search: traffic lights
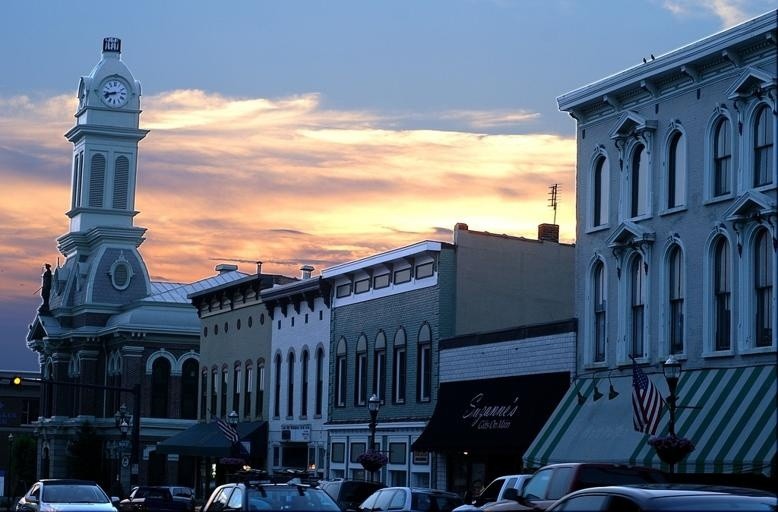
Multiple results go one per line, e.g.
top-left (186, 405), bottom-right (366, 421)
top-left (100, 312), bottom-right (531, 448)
top-left (11, 376), bottom-right (22, 387)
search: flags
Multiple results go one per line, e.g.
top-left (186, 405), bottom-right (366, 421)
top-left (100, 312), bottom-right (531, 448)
top-left (632, 358), bottom-right (662, 436)
top-left (208, 408), bottom-right (239, 445)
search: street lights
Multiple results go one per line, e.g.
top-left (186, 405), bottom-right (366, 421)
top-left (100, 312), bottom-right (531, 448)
top-left (368, 394), bottom-right (381, 480)
top-left (662, 354), bottom-right (681, 467)
top-left (228, 409), bottom-right (239, 480)
top-left (120, 420), bottom-right (129, 489)
